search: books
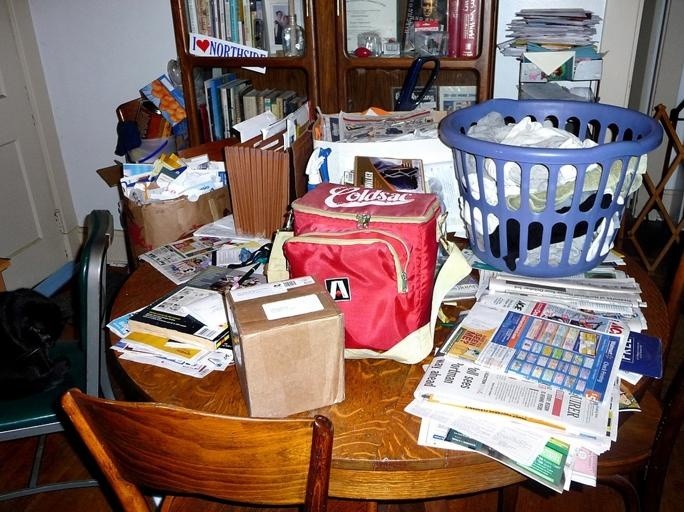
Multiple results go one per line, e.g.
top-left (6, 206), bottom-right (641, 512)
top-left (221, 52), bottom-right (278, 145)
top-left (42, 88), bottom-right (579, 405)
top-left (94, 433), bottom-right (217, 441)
top-left (194, 71), bottom-right (308, 141)
top-left (182, 0), bottom-right (266, 58)
top-left (399, 0), bottom-right (483, 60)
top-left (118, 264), bottom-right (268, 366)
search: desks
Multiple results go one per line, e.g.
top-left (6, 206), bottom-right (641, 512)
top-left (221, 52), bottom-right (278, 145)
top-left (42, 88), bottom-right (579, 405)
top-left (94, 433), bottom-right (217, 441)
top-left (108, 227), bottom-right (670, 501)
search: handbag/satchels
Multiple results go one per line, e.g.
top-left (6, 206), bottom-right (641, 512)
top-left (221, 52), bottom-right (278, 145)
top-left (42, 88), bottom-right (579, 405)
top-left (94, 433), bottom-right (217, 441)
top-left (265, 182), bottom-right (473, 367)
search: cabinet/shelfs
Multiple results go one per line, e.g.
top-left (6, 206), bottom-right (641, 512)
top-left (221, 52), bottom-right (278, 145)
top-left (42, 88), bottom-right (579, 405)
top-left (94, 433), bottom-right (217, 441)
top-left (169, 0), bottom-right (500, 145)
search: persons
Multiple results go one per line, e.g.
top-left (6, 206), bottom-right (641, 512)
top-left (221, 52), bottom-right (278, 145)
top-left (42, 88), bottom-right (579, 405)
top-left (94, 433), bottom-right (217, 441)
top-left (273, 10), bottom-right (283, 44)
top-left (283, 16), bottom-right (289, 25)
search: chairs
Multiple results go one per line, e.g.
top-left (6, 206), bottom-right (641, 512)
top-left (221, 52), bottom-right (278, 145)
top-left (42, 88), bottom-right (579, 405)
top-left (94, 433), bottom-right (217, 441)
top-left (61, 388), bottom-right (334, 512)
top-left (0, 208), bottom-right (115, 502)
